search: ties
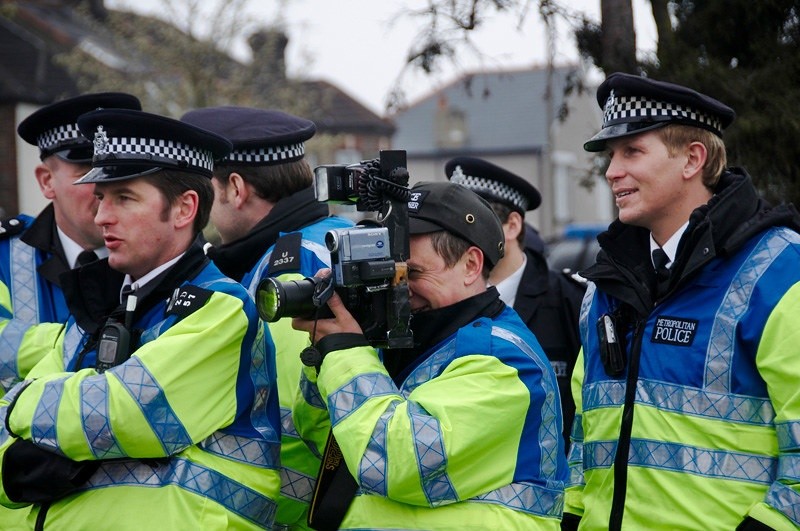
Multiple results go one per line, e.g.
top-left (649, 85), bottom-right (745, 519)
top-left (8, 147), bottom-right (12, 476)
top-left (652, 249), bottom-right (672, 295)
top-left (78, 250), bottom-right (98, 266)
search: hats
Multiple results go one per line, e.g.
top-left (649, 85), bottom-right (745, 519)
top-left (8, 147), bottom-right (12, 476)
top-left (17, 92), bottom-right (143, 164)
top-left (582, 71), bottom-right (736, 152)
top-left (409, 181), bottom-right (506, 267)
top-left (445, 156), bottom-right (542, 218)
top-left (180, 106), bottom-right (317, 165)
top-left (72, 109), bottom-right (234, 188)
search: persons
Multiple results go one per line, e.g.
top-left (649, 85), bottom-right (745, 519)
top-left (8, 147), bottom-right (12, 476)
top-left (0, 109), bottom-right (282, 531)
top-left (560, 71), bottom-right (800, 531)
top-left (0, 90), bottom-right (143, 445)
top-left (444, 157), bottom-right (584, 458)
top-left (178, 107), bottom-right (332, 531)
top-left (292, 182), bottom-right (571, 531)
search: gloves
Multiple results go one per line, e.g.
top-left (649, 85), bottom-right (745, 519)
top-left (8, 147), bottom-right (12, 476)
top-left (8, 437), bottom-right (95, 506)
